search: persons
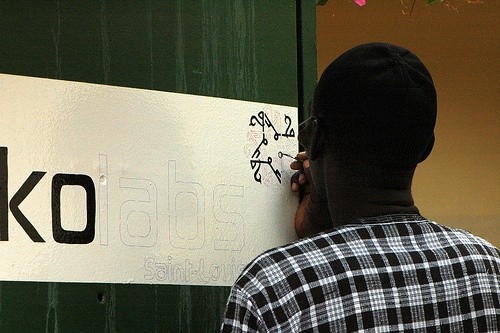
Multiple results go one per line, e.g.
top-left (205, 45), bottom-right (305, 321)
top-left (219, 42), bottom-right (500, 333)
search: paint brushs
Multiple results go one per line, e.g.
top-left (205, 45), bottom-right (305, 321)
top-left (283, 154), bottom-right (304, 163)
